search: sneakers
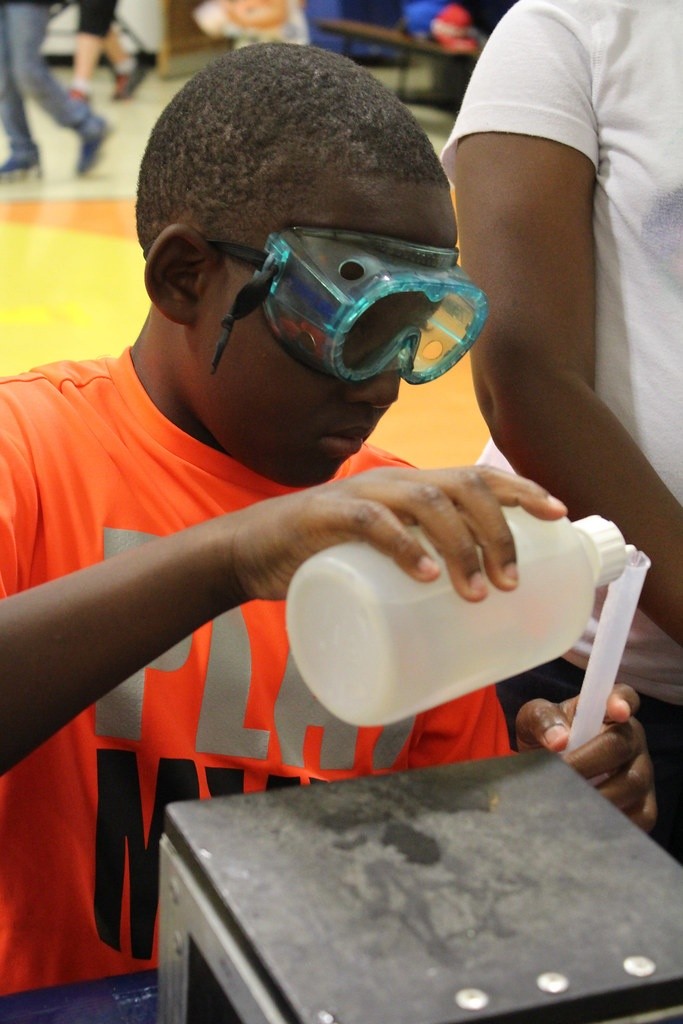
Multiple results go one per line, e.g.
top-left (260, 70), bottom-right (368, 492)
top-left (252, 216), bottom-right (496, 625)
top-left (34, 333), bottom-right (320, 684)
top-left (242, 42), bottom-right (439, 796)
top-left (76, 119), bottom-right (114, 174)
top-left (0, 147), bottom-right (40, 184)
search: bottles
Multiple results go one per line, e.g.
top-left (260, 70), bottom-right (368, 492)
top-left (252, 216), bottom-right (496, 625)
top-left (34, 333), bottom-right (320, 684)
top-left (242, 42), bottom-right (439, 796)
top-left (284, 504), bottom-right (637, 729)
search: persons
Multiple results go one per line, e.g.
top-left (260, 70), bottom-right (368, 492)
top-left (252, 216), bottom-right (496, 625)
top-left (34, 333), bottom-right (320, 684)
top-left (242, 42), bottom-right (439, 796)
top-left (0, 45), bottom-right (661, 1024)
top-left (442, 1), bottom-right (683, 861)
top-left (0, 1), bottom-right (113, 180)
top-left (66, 2), bottom-right (150, 114)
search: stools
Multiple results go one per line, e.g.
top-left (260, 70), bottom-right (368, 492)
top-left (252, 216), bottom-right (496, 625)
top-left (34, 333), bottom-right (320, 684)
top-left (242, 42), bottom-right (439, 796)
top-left (156, 749), bottom-right (683, 1024)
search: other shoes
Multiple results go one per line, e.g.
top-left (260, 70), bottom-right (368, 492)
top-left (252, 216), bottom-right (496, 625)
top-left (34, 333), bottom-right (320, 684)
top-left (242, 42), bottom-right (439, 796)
top-left (112, 53), bottom-right (156, 101)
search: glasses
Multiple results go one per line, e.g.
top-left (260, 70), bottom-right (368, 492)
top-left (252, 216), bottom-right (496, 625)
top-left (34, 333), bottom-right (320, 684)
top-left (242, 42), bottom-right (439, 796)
top-left (202, 221), bottom-right (491, 386)
